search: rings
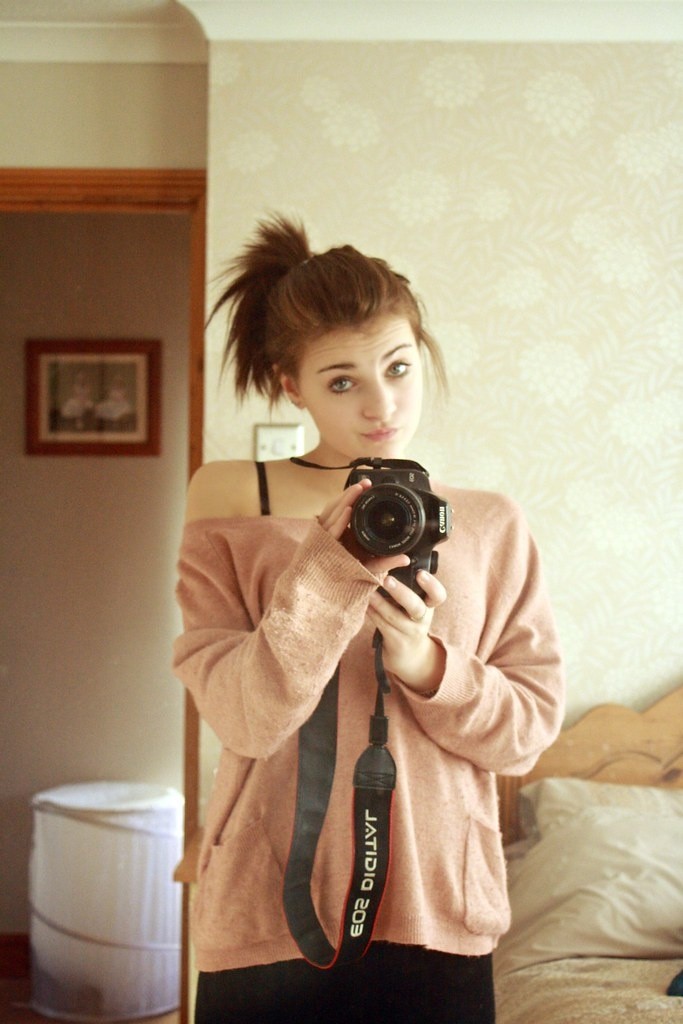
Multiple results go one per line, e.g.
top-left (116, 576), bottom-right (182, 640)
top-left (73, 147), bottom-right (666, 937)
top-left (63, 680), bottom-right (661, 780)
top-left (411, 610), bottom-right (427, 622)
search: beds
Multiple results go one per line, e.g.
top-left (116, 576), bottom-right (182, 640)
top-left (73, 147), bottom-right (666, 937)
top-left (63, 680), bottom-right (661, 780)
top-left (491, 683), bottom-right (682, 1023)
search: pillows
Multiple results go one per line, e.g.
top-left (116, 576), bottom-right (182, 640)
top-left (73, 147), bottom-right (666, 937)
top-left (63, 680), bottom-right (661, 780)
top-left (515, 777), bottom-right (682, 849)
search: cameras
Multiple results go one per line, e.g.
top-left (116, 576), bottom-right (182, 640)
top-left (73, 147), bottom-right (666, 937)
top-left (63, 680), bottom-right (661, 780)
top-left (344, 458), bottom-right (450, 604)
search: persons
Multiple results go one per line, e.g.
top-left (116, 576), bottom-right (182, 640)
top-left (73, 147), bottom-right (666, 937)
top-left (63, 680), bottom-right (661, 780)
top-left (173, 217), bottom-right (566, 1024)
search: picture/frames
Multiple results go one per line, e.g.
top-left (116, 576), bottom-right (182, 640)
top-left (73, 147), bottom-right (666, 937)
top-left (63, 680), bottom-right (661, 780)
top-left (25, 339), bottom-right (164, 456)
